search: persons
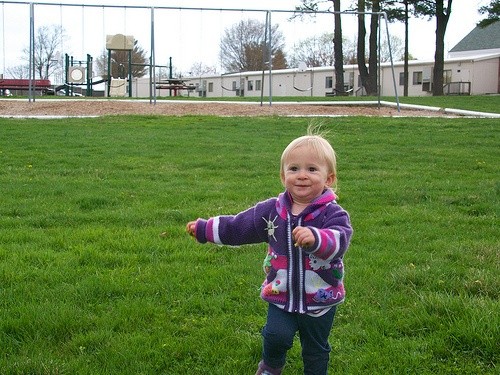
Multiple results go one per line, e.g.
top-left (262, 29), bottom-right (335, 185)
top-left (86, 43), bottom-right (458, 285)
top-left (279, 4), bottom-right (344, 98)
top-left (187, 119), bottom-right (353, 375)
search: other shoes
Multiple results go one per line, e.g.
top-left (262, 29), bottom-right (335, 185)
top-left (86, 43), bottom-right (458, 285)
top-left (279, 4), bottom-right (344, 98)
top-left (256, 360), bottom-right (283, 375)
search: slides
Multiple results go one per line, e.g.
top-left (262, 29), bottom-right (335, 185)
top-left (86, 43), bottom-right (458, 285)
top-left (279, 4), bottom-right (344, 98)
top-left (55, 83), bottom-right (66, 92)
